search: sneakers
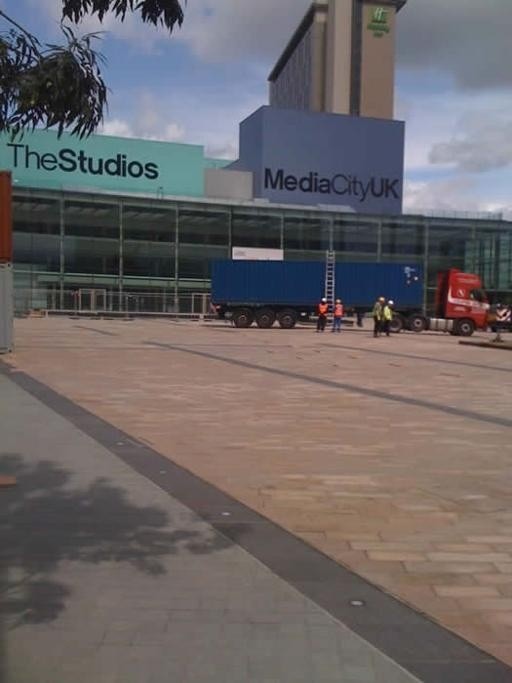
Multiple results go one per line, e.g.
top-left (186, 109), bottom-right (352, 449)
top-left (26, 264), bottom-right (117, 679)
top-left (315, 328), bottom-right (325, 333)
top-left (331, 328), bottom-right (342, 333)
top-left (373, 330), bottom-right (390, 337)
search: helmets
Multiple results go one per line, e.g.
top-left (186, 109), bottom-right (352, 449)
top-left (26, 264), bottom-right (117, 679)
top-left (387, 299), bottom-right (394, 305)
top-left (321, 297), bottom-right (328, 303)
top-left (379, 296), bottom-right (385, 301)
top-left (336, 299), bottom-right (342, 304)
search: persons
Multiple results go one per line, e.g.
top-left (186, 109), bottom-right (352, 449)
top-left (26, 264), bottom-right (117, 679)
top-left (316, 298), bottom-right (329, 330)
top-left (332, 299), bottom-right (343, 332)
top-left (380, 300), bottom-right (394, 336)
top-left (372, 297), bottom-right (385, 336)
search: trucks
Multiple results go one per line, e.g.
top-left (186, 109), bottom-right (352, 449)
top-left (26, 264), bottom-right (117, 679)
top-left (210, 259), bottom-right (490, 336)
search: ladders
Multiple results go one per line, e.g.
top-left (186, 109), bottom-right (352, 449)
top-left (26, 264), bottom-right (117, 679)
top-left (324, 249), bottom-right (335, 326)
